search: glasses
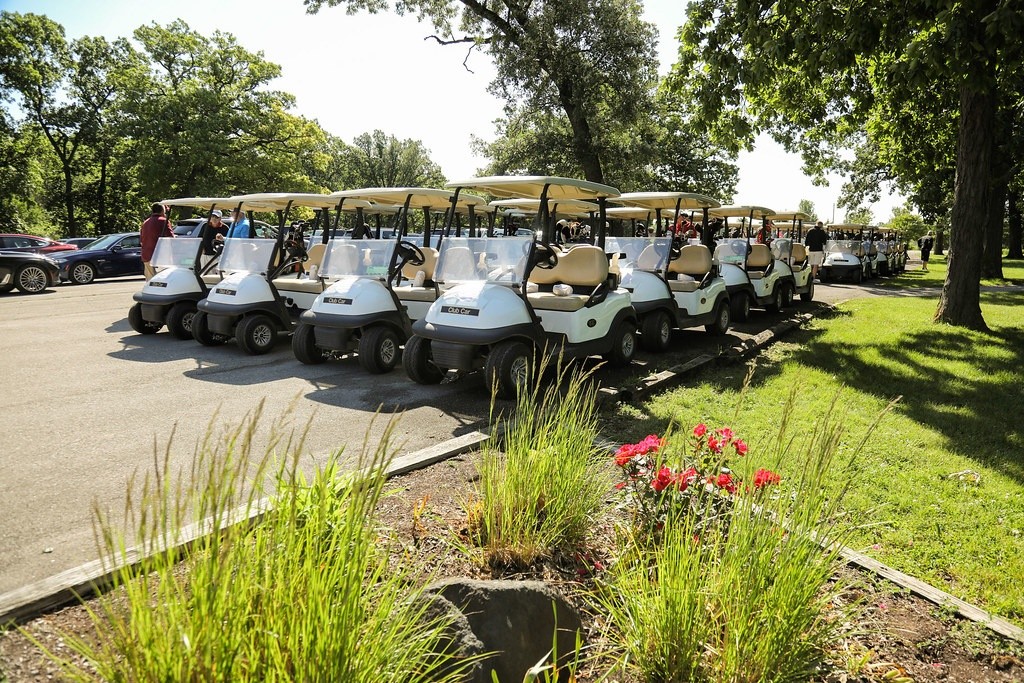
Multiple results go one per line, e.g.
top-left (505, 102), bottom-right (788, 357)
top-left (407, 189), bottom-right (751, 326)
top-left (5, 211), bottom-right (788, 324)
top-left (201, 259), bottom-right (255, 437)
top-left (211, 214), bottom-right (219, 218)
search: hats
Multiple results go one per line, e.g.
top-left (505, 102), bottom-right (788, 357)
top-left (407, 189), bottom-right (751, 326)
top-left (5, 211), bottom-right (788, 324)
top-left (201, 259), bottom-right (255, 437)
top-left (228, 208), bottom-right (247, 213)
top-left (212, 209), bottom-right (223, 217)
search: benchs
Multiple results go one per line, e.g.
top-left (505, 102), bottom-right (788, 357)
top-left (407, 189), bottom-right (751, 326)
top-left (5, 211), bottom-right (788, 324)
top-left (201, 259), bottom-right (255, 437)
top-left (197, 242), bottom-right (904, 311)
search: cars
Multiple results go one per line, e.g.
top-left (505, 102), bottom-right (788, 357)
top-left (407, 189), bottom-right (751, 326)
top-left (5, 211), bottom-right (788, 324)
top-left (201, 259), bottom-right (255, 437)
top-left (0, 249), bottom-right (62, 295)
top-left (0, 234), bottom-right (78, 254)
top-left (45, 232), bottom-right (145, 285)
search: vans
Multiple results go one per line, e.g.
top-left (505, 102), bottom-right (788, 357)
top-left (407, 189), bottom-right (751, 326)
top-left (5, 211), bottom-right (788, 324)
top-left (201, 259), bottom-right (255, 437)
top-left (172, 218), bottom-right (287, 239)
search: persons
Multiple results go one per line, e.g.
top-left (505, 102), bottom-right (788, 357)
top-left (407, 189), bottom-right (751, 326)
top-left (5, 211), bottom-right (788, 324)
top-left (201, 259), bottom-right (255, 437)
top-left (918, 230), bottom-right (934, 271)
top-left (140, 202), bottom-right (176, 284)
top-left (668, 214), bottom-right (831, 284)
top-left (196, 208), bottom-right (251, 276)
